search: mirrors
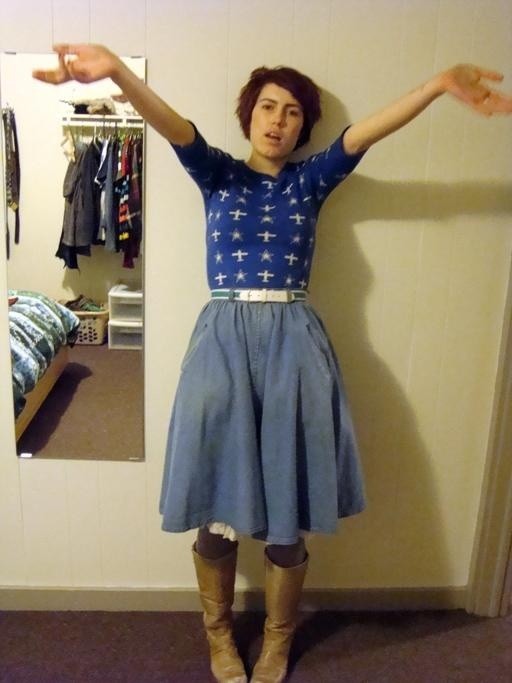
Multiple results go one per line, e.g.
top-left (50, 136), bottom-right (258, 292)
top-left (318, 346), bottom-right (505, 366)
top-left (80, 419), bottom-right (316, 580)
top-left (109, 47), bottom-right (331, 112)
top-left (0, 50), bottom-right (146, 463)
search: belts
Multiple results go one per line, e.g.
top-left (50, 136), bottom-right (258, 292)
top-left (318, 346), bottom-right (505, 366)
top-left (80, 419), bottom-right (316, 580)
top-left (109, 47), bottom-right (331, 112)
top-left (211, 289), bottom-right (308, 303)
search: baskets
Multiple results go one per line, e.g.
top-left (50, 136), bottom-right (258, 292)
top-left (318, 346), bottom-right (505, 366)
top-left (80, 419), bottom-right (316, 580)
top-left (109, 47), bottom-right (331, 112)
top-left (68, 307), bottom-right (109, 345)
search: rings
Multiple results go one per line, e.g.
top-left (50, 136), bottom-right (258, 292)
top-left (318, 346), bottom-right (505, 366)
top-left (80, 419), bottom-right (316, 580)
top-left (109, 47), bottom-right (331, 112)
top-left (482, 95), bottom-right (490, 103)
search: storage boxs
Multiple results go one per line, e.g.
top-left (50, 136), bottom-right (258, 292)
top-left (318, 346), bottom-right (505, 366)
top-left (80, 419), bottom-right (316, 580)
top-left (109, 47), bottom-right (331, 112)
top-left (108, 279), bottom-right (143, 350)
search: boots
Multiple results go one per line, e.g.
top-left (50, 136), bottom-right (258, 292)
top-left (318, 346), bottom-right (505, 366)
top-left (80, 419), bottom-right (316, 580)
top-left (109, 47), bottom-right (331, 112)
top-left (192, 541), bottom-right (247, 682)
top-left (250, 546), bottom-right (309, 683)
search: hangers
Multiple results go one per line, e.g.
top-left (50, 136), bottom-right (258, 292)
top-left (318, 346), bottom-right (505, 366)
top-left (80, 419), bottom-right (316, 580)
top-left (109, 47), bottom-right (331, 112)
top-left (67, 122), bottom-right (144, 142)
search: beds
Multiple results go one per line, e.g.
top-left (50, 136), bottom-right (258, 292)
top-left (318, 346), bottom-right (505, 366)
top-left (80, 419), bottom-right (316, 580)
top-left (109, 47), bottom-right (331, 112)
top-left (8, 288), bottom-right (81, 443)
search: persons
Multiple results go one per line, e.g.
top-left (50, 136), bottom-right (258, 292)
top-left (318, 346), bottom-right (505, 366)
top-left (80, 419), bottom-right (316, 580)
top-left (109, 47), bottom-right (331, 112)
top-left (30, 42), bottom-right (512, 683)
